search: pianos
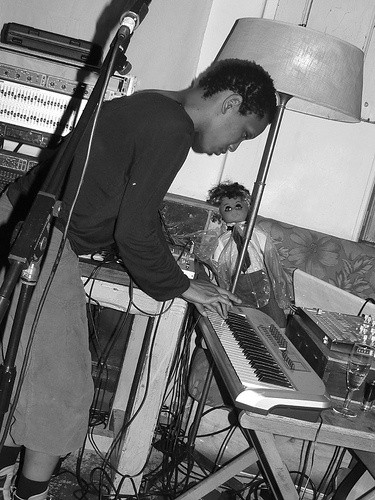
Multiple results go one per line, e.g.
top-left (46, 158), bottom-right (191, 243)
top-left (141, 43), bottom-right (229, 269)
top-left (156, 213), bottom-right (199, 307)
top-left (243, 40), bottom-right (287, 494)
top-left (191, 296), bottom-right (334, 418)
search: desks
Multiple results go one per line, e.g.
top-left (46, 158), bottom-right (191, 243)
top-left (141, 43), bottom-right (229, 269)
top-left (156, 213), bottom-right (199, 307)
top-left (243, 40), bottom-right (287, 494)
top-left (166, 321), bottom-right (375, 500)
top-left (78, 252), bottom-right (194, 500)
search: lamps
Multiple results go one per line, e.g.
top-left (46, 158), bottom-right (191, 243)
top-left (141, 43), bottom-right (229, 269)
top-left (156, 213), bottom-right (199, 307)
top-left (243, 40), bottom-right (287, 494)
top-left (138, 18), bottom-right (364, 495)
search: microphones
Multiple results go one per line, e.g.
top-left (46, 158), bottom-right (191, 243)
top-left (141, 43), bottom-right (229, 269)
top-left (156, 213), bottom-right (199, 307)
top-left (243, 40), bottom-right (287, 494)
top-left (117, 10), bottom-right (139, 39)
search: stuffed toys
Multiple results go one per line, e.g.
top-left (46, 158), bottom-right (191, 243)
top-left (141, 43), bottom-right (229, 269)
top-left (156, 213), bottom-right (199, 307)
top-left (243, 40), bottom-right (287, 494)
top-left (209, 181), bottom-right (291, 328)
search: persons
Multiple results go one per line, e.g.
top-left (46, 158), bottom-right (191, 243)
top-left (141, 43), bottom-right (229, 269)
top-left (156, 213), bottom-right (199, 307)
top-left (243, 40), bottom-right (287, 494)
top-left (0, 58), bottom-right (278, 500)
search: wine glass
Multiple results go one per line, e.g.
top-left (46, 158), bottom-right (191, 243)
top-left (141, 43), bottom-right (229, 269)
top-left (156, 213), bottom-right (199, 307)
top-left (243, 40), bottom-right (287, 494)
top-left (332, 343), bottom-right (375, 418)
